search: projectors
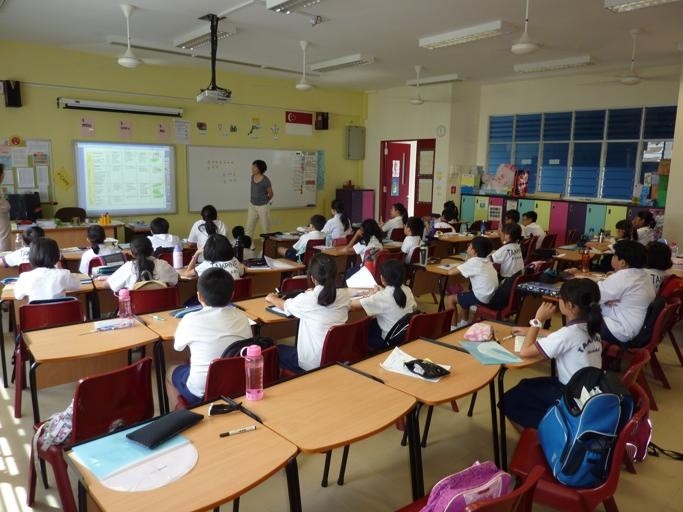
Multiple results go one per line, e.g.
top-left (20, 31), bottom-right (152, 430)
top-left (196, 89), bottom-right (232, 105)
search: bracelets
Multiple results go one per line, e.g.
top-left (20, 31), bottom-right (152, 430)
top-left (191, 255), bottom-right (198, 262)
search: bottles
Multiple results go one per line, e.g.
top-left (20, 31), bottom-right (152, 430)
top-left (588, 228), bottom-right (594, 240)
top-left (14, 232), bottom-right (23, 250)
top-left (240, 342), bottom-right (266, 401)
top-left (171, 243), bottom-right (184, 270)
top-left (111, 288), bottom-right (133, 321)
top-left (479, 217), bottom-right (486, 235)
top-left (578, 247), bottom-right (590, 273)
top-left (325, 233), bottom-right (332, 249)
top-left (105, 212), bottom-right (111, 225)
top-left (597, 231), bottom-right (604, 245)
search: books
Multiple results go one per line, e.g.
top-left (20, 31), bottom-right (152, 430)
top-left (70, 265), bottom-right (121, 284)
top-left (56, 245), bottom-right (81, 255)
top-left (246, 255), bottom-right (296, 270)
top-left (119, 240), bottom-right (135, 250)
top-left (71, 427), bottom-right (192, 482)
top-left (34, 217), bottom-right (57, 230)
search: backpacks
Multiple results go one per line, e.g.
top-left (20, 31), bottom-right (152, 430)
top-left (524, 259), bottom-right (546, 275)
top-left (625, 414), bottom-right (683, 474)
top-left (613, 295), bottom-right (666, 372)
top-left (133, 270), bottom-right (167, 291)
top-left (418, 460), bottom-right (516, 512)
top-left (385, 311), bottom-right (427, 347)
top-left (488, 263), bottom-right (536, 310)
top-left (219, 336), bottom-right (273, 358)
top-left (31, 398), bottom-right (73, 494)
top-left (537, 366), bottom-right (636, 489)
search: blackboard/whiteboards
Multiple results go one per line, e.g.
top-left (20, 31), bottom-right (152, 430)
top-left (187, 146), bottom-right (319, 214)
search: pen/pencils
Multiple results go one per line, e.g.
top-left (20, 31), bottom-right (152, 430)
top-left (503, 333), bottom-right (514, 341)
top-left (149, 315), bottom-right (165, 321)
top-left (220, 424), bottom-right (256, 437)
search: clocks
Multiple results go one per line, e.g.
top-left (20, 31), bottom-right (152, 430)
top-left (435, 125), bottom-right (446, 137)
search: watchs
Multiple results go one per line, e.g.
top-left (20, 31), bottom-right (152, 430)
top-left (529, 319), bottom-right (544, 329)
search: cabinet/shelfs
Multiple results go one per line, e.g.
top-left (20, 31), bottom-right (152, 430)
top-left (334, 188), bottom-right (374, 220)
top-left (459, 193), bottom-right (664, 251)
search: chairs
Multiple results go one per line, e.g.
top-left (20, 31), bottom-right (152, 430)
top-left (55, 207), bottom-right (85, 221)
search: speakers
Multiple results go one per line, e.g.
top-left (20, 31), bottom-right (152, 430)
top-left (3, 80), bottom-right (21, 107)
top-left (314, 111), bottom-right (329, 130)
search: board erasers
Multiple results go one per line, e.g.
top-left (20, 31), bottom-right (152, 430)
top-left (306, 203), bottom-right (316, 207)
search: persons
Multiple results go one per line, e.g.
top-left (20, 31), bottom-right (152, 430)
top-left (236, 235), bottom-right (257, 259)
top-left (166, 265), bottom-right (255, 409)
top-left (585, 212), bottom-right (674, 345)
top-left (0, 161), bottom-right (13, 253)
top-left (100, 234), bottom-right (180, 316)
top-left (264, 254), bottom-right (353, 378)
top-left (493, 276), bottom-right (606, 438)
top-left (146, 218), bottom-right (182, 252)
top-left (231, 226), bottom-right (256, 251)
top-left (275, 198), bottom-right (547, 376)
top-left (79, 223), bottom-right (128, 274)
top-left (0, 225), bottom-right (46, 266)
top-left (186, 204), bottom-right (228, 251)
top-left (184, 234), bottom-right (249, 281)
top-left (243, 158), bottom-right (275, 256)
top-left (12, 238), bottom-right (82, 303)
